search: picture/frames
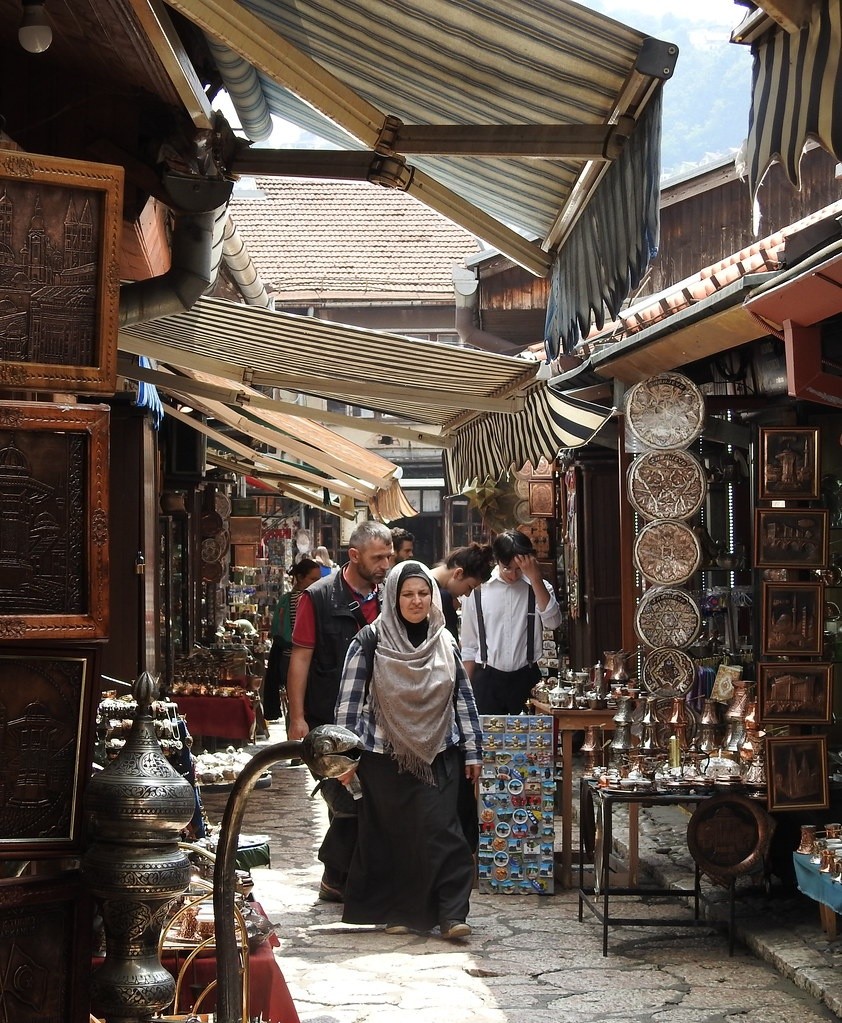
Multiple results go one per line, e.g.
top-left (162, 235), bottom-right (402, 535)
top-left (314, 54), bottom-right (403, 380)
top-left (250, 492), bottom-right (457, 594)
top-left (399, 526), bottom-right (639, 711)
top-left (0, 148), bottom-right (125, 1023)
top-left (340, 507), bottom-right (368, 546)
top-left (752, 428), bottom-right (834, 811)
top-left (528, 456), bottom-right (555, 518)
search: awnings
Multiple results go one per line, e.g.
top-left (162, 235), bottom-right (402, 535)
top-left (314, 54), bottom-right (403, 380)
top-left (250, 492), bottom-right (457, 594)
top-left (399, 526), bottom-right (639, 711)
top-left (156, 362), bottom-right (419, 523)
top-left (165, 0), bottom-right (679, 365)
top-left (730, 0), bottom-right (841, 199)
top-left (114, 297), bottom-right (615, 497)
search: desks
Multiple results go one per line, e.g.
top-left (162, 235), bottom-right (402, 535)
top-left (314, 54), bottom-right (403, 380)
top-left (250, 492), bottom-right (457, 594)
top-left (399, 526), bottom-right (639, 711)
top-left (791, 851), bottom-right (842, 937)
top-left (93, 901), bottom-right (299, 1023)
top-left (532, 698), bottom-right (750, 956)
top-left (168, 694), bottom-right (255, 753)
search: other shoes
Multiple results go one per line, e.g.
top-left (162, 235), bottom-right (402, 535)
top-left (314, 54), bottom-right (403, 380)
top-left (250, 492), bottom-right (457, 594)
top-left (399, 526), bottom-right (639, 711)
top-left (441, 919), bottom-right (473, 939)
top-left (384, 923), bottom-right (410, 935)
top-left (319, 875), bottom-right (345, 902)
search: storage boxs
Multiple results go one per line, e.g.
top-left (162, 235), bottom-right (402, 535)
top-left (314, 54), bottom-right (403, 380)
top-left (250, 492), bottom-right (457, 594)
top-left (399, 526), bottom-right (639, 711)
top-left (230, 498), bottom-right (262, 566)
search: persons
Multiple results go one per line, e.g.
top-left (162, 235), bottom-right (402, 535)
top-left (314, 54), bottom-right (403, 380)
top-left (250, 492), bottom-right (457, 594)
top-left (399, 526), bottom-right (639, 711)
top-left (314, 546), bottom-right (333, 577)
top-left (277, 558), bottom-right (321, 766)
top-left (430, 543), bottom-right (493, 641)
top-left (338, 560), bottom-right (484, 940)
top-left (461, 530), bottom-right (563, 715)
top-left (388, 528), bottom-right (415, 569)
top-left (288, 521), bottom-right (392, 903)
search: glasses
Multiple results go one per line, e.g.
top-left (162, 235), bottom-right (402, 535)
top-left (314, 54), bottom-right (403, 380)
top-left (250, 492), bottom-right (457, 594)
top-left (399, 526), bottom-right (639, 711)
top-left (499, 566), bottom-right (523, 574)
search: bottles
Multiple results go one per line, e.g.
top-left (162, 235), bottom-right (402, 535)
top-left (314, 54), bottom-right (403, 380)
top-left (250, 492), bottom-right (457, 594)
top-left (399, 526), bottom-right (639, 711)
top-left (532, 650), bottom-right (768, 787)
top-left (347, 775), bottom-right (364, 800)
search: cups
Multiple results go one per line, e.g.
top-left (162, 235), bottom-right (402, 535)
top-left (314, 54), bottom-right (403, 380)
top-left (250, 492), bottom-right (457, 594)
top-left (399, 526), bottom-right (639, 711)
top-left (796, 824), bottom-right (842, 882)
